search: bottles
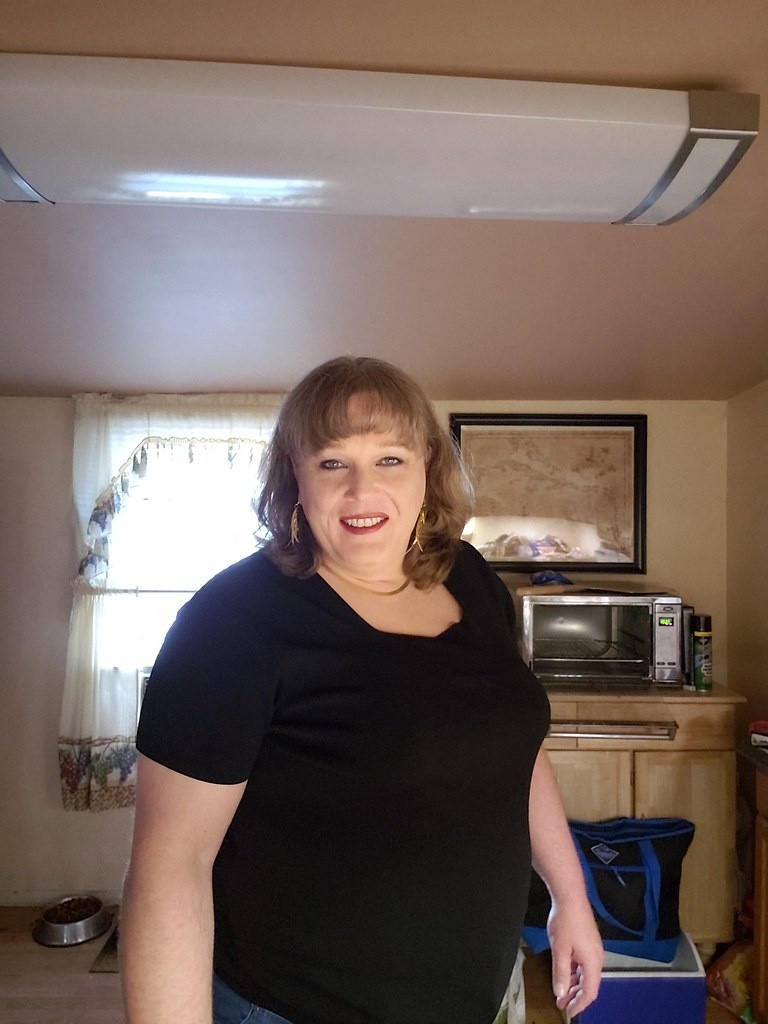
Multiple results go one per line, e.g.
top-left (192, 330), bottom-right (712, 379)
top-left (694, 616), bottom-right (714, 692)
top-left (682, 607), bottom-right (695, 685)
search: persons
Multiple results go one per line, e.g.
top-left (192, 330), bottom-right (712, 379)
top-left (118, 356), bottom-right (604, 1024)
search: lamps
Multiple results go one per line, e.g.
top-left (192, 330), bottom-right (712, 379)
top-left (0, 52), bottom-right (758, 228)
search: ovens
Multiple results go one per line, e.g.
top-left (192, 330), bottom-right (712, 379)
top-left (522, 595), bottom-right (683, 689)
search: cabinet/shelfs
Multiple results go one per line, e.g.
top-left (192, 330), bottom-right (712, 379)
top-left (529, 683), bottom-right (749, 967)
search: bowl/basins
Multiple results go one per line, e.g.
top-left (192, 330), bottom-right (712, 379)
top-left (33, 895), bottom-right (112, 947)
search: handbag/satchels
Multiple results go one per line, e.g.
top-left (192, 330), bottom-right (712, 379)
top-left (521, 816), bottom-right (696, 964)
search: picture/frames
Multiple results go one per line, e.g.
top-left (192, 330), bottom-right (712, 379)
top-left (449, 411), bottom-right (647, 575)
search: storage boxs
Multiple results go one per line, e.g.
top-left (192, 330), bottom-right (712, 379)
top-left (565, 928), bottom-right (706, 1024)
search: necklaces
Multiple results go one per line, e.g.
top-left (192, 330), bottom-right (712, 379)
top-left (320, 560), bottom-right (413, 596)
